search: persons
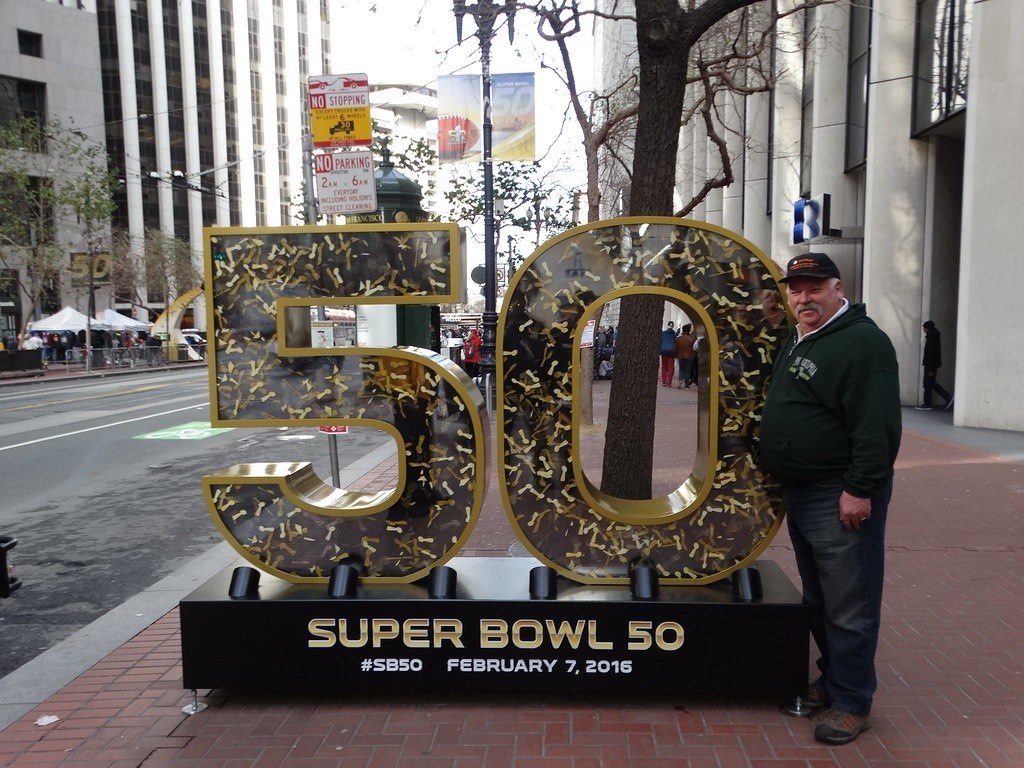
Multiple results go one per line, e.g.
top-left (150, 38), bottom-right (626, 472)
top-left (659, 321), bottom-right (699, 389)
top-left (755, 253), bottom-right (904, 745)
top-left (593, 325), bottom-right (619, 380)
top-left (914, 320), bottom-right (954, 410)
top-left (185, 336), bottom-right (199, 345)
top-left (441, 326), bottom-right (484, 379)
top-left (0, 331), bottom-right (163, 367)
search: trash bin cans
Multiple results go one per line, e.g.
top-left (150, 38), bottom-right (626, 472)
top-left (177, 343), bottom-right (188, 363)
top-left (449, 344), bottom-right (464, 366)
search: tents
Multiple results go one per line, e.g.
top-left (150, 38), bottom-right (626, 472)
top-left (26, 305), bottom-right (112, 335)
top-left (91, 307), bottom-right (155, 334)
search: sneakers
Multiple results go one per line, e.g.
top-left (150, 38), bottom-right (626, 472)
top-left (815, 707), bottom-right (871, 743)
top-left (804, 675), bottom-right (825, 707)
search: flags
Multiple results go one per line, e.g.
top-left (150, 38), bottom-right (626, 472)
top-left (437, 72), bottom-right (535, 166)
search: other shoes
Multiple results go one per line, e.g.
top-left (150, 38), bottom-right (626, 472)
top-left (915, 404), bottom-right (933, 410)
top-left (685, 386), bottom-right (691, 389)
top-left (662, 383), bottom-right (671, 387)
top-left (945, 396), bottom-right (953, 409)
top-left (677, 381), bottom-right (682, 389)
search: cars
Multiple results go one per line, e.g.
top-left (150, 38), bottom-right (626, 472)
top-left (183, 334), bottom-right (206, 343)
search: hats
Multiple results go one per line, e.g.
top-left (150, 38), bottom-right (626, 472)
top-left (778, 253), bottom-right (840, 283)
top-left (668, 321), bottom-right (674, 328)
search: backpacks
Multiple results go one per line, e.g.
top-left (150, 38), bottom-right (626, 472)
top-left (463, 338), bottom-right (474, 355)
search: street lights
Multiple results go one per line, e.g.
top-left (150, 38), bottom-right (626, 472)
top-left (451, 0), bottom-right (517, 419)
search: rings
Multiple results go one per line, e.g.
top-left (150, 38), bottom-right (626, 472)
top-left (861, 517), bottom-right (866, 521)
